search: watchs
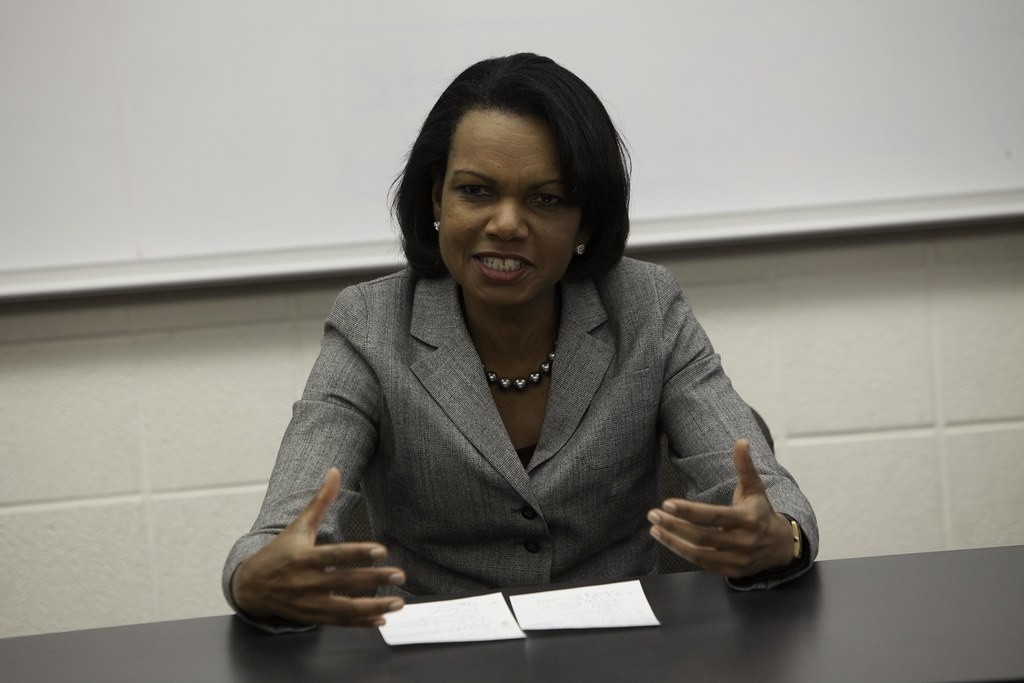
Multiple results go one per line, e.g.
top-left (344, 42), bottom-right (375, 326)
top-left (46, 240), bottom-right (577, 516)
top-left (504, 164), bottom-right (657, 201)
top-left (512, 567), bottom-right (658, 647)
top-left (784, 513), bottom-right (802, 558)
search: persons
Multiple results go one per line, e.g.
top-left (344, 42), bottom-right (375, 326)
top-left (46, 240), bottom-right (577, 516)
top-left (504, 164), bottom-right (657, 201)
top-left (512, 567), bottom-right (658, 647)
top-left (222, 53), bottom-right (819, 633)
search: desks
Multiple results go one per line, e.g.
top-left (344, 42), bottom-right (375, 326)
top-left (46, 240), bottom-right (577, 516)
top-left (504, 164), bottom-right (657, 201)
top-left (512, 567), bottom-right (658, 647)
top-left (0, 546), bottom-right (1024, 683)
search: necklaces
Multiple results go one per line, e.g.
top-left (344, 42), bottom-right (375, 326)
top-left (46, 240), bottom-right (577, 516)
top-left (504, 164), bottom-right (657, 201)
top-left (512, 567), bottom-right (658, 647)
top-left (481, 350), bottom-right (558, 390)
